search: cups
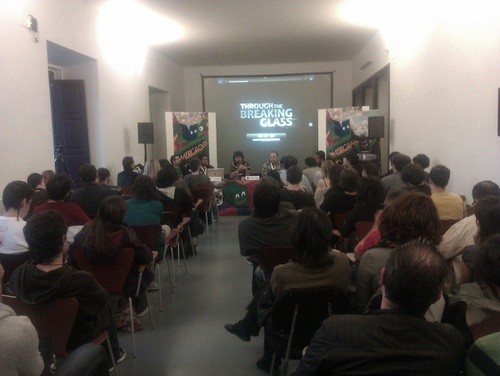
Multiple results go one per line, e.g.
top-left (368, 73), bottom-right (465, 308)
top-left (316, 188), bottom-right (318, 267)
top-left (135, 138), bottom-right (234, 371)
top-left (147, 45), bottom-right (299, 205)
top-left (241, 176), bottom-right (244, 180)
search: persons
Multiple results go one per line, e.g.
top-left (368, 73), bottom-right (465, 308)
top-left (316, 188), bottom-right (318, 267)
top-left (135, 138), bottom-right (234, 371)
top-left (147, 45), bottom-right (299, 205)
top-left (224, 150), bottom-right (500, 376)
top-left (0, 155), bottom-right (216, 376)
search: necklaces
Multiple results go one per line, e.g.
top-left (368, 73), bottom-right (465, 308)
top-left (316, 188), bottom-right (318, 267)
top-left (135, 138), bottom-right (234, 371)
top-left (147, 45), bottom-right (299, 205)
top-left (37, 263), bottom-right (64, 267)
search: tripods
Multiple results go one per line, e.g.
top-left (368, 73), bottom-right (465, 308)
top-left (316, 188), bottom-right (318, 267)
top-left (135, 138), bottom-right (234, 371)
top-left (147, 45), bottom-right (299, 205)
top-left (55, 144), bottom-right (78, 190)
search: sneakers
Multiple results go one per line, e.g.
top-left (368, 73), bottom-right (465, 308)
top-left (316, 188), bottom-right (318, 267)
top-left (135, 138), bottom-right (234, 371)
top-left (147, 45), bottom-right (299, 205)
top-left (224, 321), bottom-right (250, 341)
top-left (108, 347), bottom-right (127, 371)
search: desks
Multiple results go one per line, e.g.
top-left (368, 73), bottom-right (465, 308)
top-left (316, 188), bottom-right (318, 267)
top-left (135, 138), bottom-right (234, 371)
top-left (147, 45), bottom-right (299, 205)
top-left (211, 176), bottom-right (261, 216)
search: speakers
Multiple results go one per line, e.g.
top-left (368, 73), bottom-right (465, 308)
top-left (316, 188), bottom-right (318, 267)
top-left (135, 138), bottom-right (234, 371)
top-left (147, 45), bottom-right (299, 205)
top-left (138, 122), bottom-right (154, 143)
top-left (368, 116), bottom-right (384, 138)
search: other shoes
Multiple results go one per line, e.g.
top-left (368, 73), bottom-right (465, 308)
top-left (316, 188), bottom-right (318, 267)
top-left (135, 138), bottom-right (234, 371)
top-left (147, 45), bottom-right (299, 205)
top-left (133, 304), bottom-right (151, 316)
top-left (147, 281), bottom-right (159, 290)
top-left (256, 358), bottom-right (282, 376)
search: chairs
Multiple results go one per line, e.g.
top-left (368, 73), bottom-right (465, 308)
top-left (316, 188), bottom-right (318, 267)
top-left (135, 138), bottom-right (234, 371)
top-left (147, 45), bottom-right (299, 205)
top-left (0, 186), bottom-right (500, 376)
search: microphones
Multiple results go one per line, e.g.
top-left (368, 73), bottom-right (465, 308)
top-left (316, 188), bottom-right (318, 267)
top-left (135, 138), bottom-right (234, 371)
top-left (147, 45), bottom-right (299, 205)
top-left (238, 161), bottom-right (239, 167)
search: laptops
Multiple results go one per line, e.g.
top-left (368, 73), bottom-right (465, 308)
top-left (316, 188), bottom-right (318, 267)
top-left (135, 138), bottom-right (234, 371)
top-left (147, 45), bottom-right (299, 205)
top-left (205, 168), bottom-right (224, 181)
